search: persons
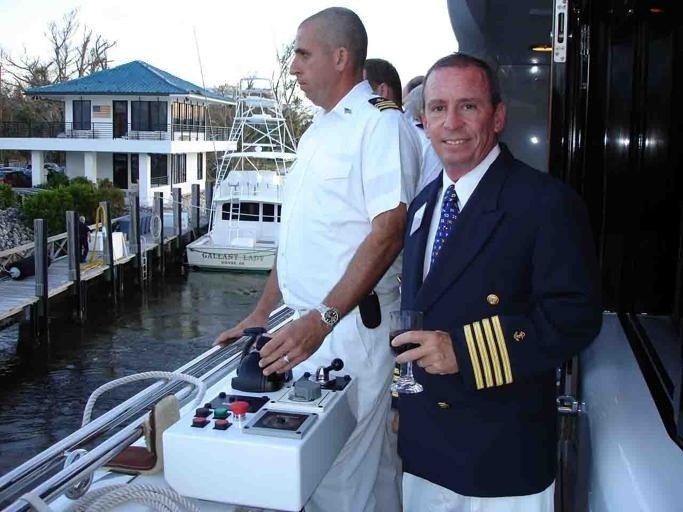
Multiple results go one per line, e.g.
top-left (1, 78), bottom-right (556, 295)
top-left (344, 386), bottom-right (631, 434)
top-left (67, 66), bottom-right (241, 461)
top-left (208, 5), bottom-right (425, 511)
top-left (77, 213), bottom-right (92, 263)
top-left (362, 56), bottom-right (442, 195)
top-left (386, 52), bottom-right (606, 511)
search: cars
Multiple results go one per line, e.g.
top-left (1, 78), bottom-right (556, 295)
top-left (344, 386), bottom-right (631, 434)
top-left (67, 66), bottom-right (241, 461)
top-left (0, 162), bottom-right (62, 181)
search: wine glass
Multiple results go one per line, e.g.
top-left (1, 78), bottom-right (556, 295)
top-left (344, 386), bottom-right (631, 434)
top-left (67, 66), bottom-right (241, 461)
top-left (386, 308), bottom-right (425, 395)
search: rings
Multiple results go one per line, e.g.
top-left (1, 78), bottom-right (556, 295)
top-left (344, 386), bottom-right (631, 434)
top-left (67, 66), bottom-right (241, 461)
top-left (418, 360), bottom-right (426, 368)
top-left (282, 354), bottom-right (290, 364)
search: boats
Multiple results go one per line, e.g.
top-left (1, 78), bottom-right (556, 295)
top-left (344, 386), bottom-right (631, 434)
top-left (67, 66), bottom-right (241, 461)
top-left (186, 77), bottom-right (297, 272)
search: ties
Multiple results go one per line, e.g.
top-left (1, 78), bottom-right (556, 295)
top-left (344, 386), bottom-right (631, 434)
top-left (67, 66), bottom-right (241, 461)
top-left (430, 183), bottom-right (461, 270)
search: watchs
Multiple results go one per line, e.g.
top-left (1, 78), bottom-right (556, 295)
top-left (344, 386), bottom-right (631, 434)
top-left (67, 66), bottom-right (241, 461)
top-left (316, 302), bottom-right (341, 328)
